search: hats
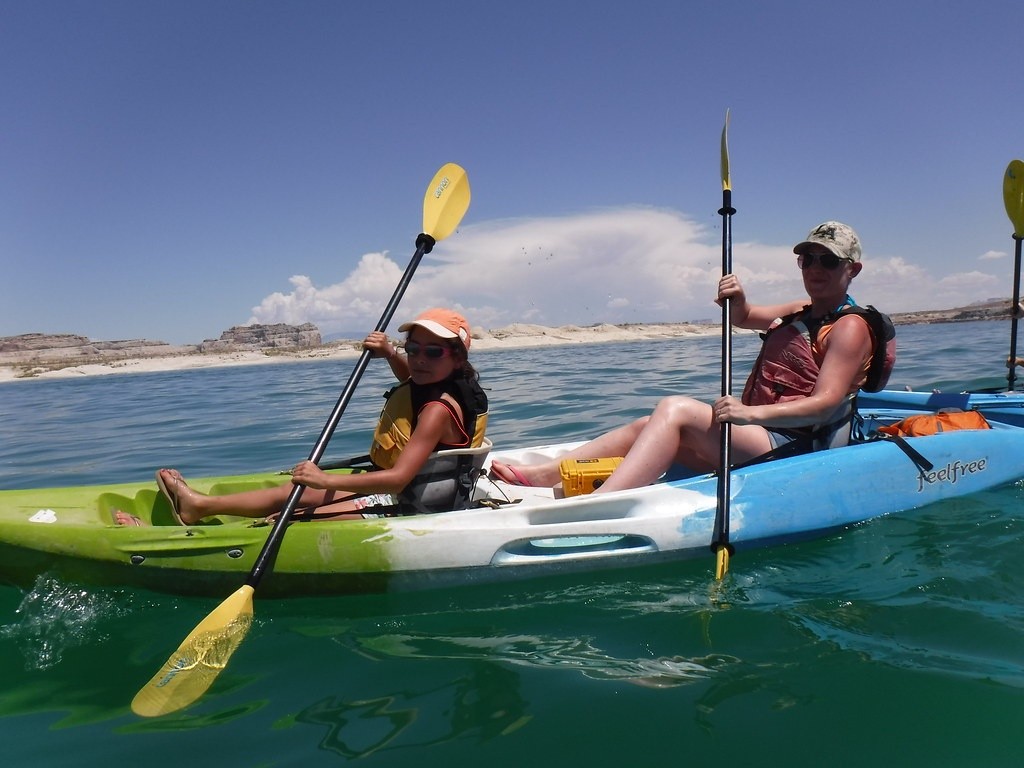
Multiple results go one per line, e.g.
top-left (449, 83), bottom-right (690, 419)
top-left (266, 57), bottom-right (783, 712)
top-left (398, 308), bottom-right (471, 352)
top-left (793, 221), bottom-right (861, 262)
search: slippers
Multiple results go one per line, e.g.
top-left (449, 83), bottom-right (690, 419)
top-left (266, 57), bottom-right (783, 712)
top-left (111, 509), bottom-right (139, 526)
top-left (156, 469), bottom-right (196, 526)
top-left (489, 464), bottom-right (531, 487)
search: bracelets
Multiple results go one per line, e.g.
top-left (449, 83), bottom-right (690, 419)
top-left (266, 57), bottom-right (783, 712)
top-left (387, 345), bottom-right (397, 360)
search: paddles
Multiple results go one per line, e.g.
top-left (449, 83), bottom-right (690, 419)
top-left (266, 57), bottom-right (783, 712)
top-left (1001, 159), bottom-right (1024, 392)
top-left (701, 105), bottom-right (738, 650)
top-left (130, 162), bottom-right (471, 718)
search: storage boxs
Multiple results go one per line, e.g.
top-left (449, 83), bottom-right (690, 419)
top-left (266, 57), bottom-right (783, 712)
top-left (559, 456), bottom-right (624, 498)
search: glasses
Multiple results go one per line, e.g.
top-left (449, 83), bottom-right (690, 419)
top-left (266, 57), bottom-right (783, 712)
top-left (797, 253), bottom-right (846, 270)
top-left (405, 341), bottom-right (451, 359)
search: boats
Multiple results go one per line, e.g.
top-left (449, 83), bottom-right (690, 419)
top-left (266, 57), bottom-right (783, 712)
top-left (855, 386), bottom-right (1024, 428)
top-left (0, 406), bottom-right (1024, 586)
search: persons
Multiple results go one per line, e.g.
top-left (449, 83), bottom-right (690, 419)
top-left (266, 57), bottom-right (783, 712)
top-left (107, 305), bottom-right (491, 529)
top-left (490, 222), bottom-right (878, 495)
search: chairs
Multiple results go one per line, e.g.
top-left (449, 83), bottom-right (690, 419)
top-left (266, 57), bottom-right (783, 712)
top-left (812, 391), bottom-right (858, 451)
top-left (350, 437), bottom-right (493, 518)
